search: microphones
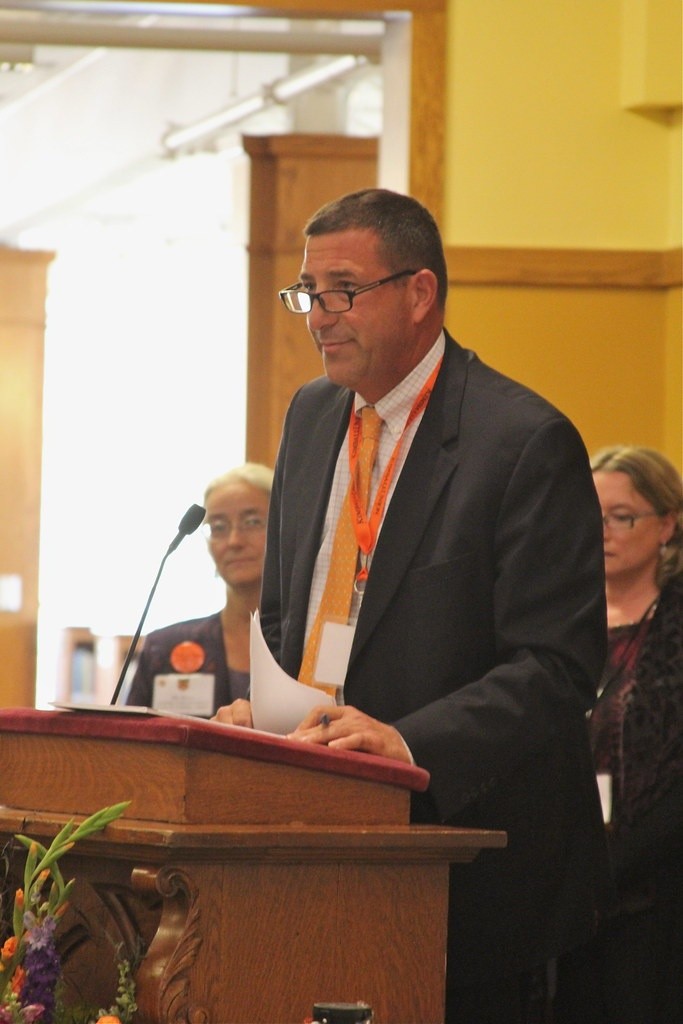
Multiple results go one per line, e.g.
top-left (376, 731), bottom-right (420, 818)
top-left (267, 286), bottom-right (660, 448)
top-left (111, 503), bottom-right (205, 705)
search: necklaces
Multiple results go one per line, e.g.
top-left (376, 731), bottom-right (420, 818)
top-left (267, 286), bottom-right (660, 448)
top-left (585, 594), bottom-right (660, 719)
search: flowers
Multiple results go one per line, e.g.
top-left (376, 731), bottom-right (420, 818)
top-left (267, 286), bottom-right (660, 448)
top-left (0, 800), bottom-right (132, 1022)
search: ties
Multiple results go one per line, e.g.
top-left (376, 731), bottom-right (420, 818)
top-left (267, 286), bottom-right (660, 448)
top-left (297, 406), bottom-right (383, 697)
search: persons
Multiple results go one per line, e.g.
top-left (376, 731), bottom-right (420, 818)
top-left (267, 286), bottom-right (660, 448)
top-left (590, 447), bottom-right (683, 1024)
top-left (125, 462), bottom-right (276, 730)
top-left (206, 188), bottom-right (609, 1024)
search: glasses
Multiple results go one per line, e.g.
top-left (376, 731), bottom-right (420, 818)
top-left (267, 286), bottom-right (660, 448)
top-left (201, 515), bottom-right (267, 543)
top-left (602, 510), bottom-right (660, 531)
top-left (278, 267), bottom-right (417, 314)
